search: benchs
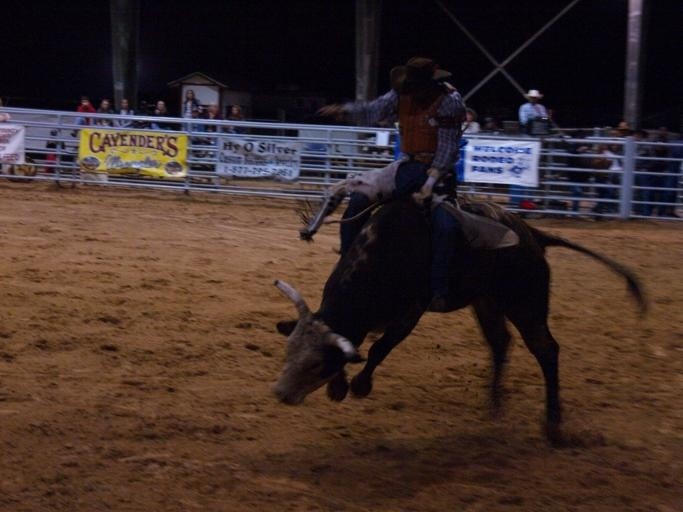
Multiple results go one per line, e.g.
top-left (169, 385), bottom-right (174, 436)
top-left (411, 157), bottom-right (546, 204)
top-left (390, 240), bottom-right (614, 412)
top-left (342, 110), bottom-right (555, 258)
top-left (0, 108), bottom-right (101, 153)
top-left (351, 124), bottom-right (609, 216)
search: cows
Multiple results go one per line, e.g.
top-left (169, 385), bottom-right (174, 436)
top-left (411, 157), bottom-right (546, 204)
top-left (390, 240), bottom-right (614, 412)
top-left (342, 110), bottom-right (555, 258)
top-left (270, 193), bottom-right (650, 451)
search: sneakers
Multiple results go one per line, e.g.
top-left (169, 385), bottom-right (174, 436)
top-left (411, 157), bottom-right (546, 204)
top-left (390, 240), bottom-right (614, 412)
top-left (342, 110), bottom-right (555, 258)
top-left (428, 291), bottom-right (448, 311)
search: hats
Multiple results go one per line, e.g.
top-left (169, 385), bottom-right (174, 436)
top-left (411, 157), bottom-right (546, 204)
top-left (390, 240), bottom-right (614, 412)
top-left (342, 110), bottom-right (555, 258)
top-left (522, 88), bottom-right (545, 99)
top-left (614, 121), bottom-right (636, 132)
top-left (389, 56), bottom-right (453, 92)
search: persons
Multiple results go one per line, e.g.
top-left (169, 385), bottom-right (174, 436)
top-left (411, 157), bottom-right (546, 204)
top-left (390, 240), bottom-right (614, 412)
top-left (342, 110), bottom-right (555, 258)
top-left (317, 59), bottom-right (466, 311)
top-left (77, 88), bottom-right (250, 174)
top-left (461, 90), bottom-right (683, 216)
